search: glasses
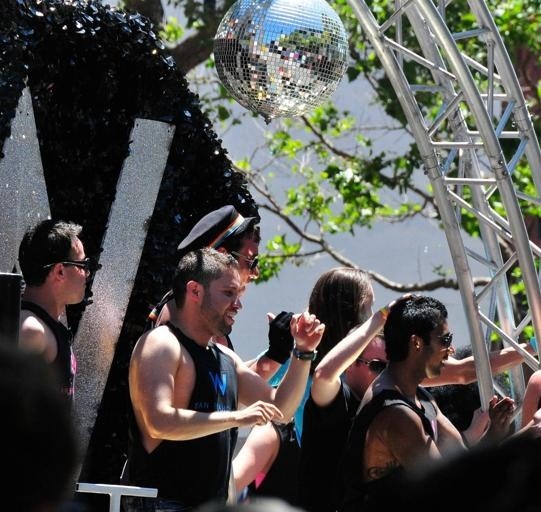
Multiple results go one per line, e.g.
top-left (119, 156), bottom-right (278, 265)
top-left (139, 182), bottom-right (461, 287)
top-left (431, 329), bottom-right (454, 348)
top-left (43, 257), bottom-right (92, 272)
top-left (233, 249), bottom-right (262, 271)
top-left (357, 356), bottom-right (388, 375)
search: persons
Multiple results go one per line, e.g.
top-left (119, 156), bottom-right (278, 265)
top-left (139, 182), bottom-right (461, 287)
top-left (0, 220), bottom-right (92, 512)
top-left (129, 206), bottom-right (541, 511)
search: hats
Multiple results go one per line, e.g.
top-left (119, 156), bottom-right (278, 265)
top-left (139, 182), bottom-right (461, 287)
top-left (177, 206), bottom-right (258, 253)
